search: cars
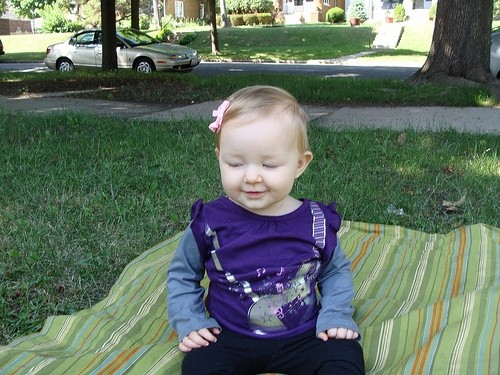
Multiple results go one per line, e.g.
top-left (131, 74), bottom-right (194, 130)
top-left (44, 27), bottom-right (202, 74)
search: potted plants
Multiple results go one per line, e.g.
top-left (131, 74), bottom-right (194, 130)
top-left (384, 10), bottom-right (393, 23)
top-left (350, 3), bottom-right (368, 27)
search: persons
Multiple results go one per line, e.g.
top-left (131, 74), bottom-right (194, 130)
top-left (166, 85), bottom-right (365, 375)
